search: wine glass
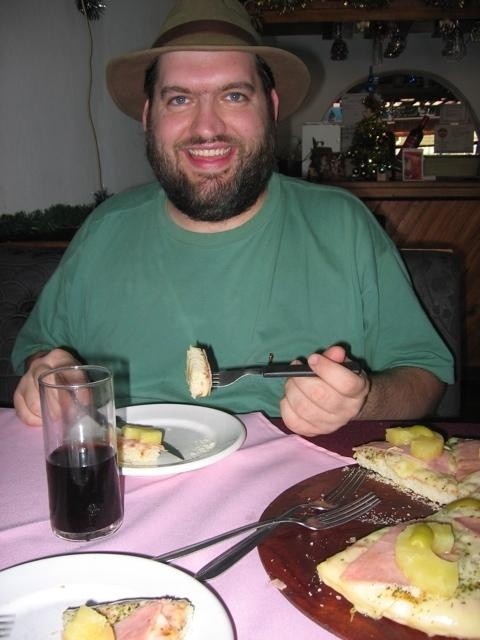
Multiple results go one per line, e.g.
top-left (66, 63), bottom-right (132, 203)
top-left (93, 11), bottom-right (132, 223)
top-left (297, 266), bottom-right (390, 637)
top-left (371, 20), bottom-right (384, 65)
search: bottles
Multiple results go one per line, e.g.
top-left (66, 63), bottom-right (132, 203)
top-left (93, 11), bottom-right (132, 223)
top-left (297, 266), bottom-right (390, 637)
top-left (396, 114), bottom-right (429, 162)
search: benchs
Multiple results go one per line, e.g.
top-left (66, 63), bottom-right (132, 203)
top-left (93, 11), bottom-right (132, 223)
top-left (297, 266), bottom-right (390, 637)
top-left (0, 241), bottom-right (468, 423)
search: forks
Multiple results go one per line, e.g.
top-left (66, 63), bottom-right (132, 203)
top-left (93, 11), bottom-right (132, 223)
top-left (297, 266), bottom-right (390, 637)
top-left (0, 610), bottom-right (16, 638)
top-left (211, 361), bottom-right (363, 389)
top-left (152, 490), bottom-right (384, 562)
top-left (193, 466), bottom-right (365, 581)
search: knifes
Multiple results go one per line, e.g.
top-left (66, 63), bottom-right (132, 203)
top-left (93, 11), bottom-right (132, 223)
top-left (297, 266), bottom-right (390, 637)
top-left (79, 400), bottom-right (185, 460)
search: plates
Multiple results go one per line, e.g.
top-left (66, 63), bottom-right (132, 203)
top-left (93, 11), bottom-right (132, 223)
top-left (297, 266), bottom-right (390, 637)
top-left (65, 402), bottom-right (247, 477)
top-left (257, 463), bottom-right (480, 639)
top-left (0, 554), bottom-right (236, 640)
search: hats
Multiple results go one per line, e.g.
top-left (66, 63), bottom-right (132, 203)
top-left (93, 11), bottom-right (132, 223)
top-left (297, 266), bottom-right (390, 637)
top-left (106, 0), bottom-right (312, 125)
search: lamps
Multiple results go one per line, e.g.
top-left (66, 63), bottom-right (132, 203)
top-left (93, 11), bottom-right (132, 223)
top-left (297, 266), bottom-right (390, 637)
top-left (330, 18), bottom-right (467, 65)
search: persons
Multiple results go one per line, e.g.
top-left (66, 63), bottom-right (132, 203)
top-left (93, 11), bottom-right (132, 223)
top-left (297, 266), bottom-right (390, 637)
top-left (11, 0), bottom-right (450, 437)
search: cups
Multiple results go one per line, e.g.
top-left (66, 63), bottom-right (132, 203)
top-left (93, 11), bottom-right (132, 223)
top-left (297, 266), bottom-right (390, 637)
top-left (34, 364), bottom-right (124, 544)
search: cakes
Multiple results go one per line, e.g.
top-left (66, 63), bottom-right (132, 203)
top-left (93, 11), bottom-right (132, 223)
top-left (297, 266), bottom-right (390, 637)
top-left (184, 347), bottom-right (214, 399)
top-left (115, 424), bottom-right (163, 464)
top-left (61, 597), bottom-right (193, 640)
top-left (317, 424), bottom-right (480, 638)
